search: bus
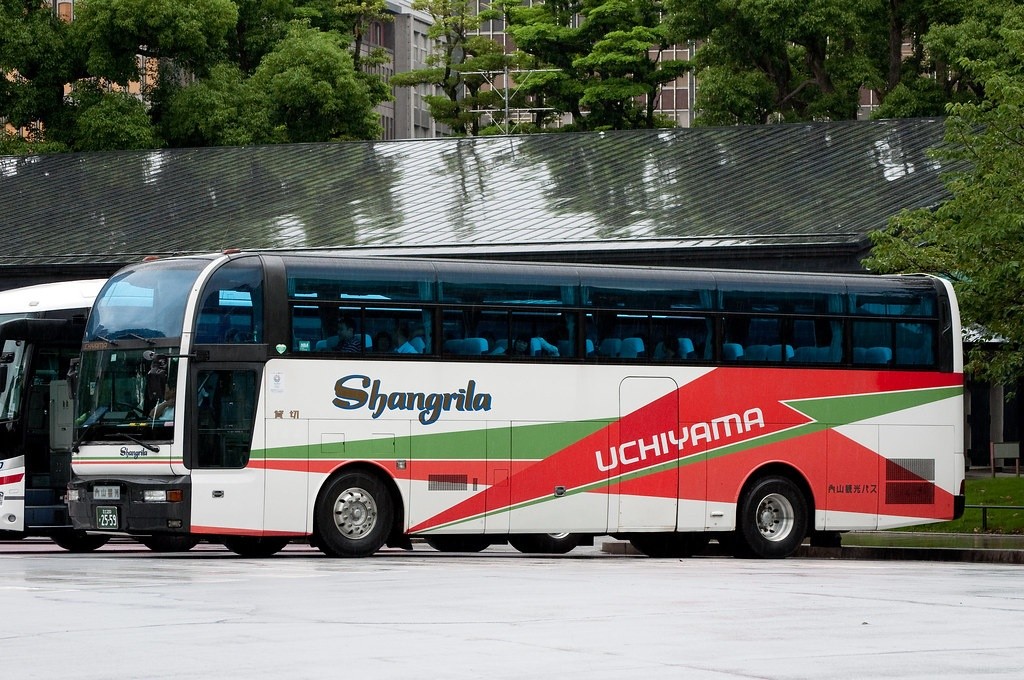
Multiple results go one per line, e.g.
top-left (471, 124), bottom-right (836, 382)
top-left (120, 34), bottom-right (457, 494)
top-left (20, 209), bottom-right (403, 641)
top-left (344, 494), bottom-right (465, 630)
top-left (0, 253), bottom-right (967, 560)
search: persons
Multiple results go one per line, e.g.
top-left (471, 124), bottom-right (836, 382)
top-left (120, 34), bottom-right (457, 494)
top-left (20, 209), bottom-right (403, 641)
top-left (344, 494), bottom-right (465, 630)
top-left (148, 379), bottom-right (176, 421)
top-left (314, 316), bottom-right (680, 359)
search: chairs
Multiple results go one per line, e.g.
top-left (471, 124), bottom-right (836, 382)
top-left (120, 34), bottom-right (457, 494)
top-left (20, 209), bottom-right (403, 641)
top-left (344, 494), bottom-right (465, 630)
top-left (311, 331), bottom-right (924, 364)
